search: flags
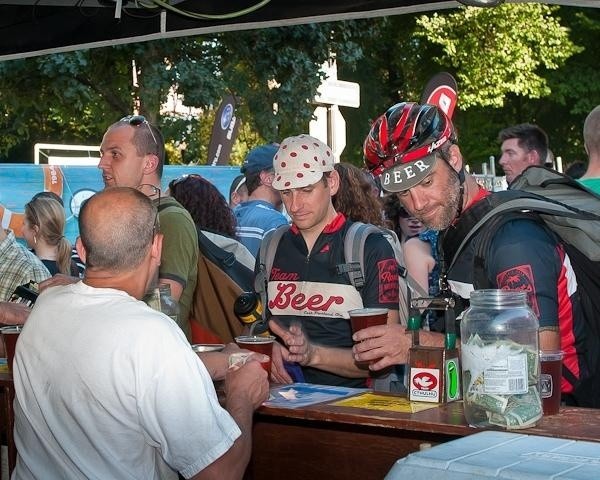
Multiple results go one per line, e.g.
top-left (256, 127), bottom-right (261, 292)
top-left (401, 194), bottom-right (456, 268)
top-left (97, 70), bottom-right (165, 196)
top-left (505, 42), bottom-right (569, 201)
top-left (421, 73), bottom-right (457, 120)
top-left (207, 97), bottom-right (242, 166)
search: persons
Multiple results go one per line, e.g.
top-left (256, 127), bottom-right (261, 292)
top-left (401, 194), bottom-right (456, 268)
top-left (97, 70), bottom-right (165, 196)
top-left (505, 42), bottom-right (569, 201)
top-left (9, 186), bottom-right (270, 477)
top-left (0, 101), bottom-right (599, 407)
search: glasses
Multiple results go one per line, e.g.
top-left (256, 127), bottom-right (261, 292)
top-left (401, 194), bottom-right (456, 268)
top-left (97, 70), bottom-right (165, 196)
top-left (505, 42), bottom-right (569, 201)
top-left (121, 116), bottom-right (157, 144)
top-left (135, 184), bottom-right (160, 244)
top-left (171, 173), bottom-right (199, 185)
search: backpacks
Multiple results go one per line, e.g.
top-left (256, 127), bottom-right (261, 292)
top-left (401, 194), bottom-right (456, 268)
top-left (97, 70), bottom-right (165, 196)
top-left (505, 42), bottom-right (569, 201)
top-left (191, 225), bottom-right (256, 344)
top-left (344, 222), bottom-right (419, 393)
top-left (504, 164), bottom-right (600, 318)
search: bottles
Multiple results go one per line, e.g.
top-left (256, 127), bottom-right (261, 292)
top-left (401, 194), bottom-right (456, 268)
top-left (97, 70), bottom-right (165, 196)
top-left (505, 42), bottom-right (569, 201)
top-left (456, 286), bottom-right (546, 432)
top-left (142, 281), bottom-right (180, 327)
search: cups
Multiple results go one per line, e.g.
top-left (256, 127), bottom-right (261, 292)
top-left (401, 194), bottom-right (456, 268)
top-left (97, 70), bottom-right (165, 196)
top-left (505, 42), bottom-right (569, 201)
top-left (348, 307), bottom-right (392, 366)
top-left (0, 324), bottom-right (22, 370)
top-left (539, 350), bottom-right (565, 416)
top-left (234, 335), bottom-right (276, 386)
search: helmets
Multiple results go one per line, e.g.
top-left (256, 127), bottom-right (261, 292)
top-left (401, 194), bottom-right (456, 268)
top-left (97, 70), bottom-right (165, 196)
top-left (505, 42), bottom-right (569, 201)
top-left (364, 102), bottom-right (454, 179)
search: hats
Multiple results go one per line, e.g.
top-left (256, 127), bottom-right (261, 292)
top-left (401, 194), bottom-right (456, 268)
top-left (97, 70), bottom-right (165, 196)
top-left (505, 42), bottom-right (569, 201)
top-left (241, 145), bottom-right (278, 175)
top-left (376, 154), bottom-right (436, 193)
top-left (271, 134), bottom-right (335, 190)
